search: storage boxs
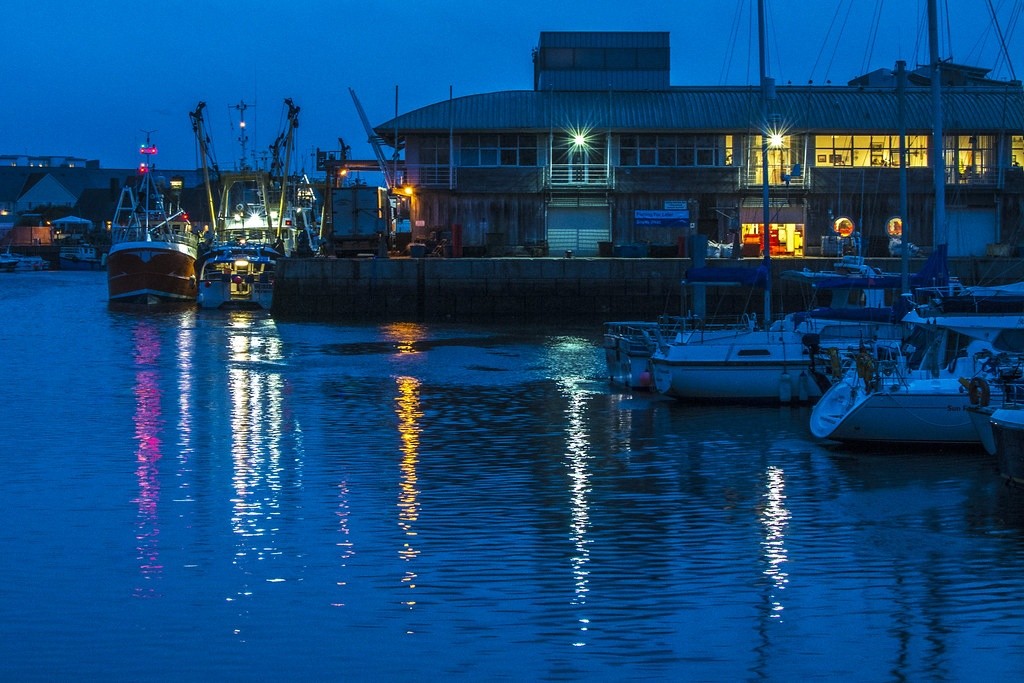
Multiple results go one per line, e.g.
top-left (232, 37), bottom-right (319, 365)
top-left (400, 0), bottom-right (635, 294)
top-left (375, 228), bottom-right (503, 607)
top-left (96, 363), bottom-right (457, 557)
top-left (648, 243), bottom-right (679, 257)
top-left (614, 242), bottom-right (647, 258)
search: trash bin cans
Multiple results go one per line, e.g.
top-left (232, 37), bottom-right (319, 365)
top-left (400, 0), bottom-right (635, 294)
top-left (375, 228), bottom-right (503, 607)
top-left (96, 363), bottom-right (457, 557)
top-left (411, 244), bottom-right (426, 258)
top-left (744, 234), bottom-right (761, 256)
top-left (615, 243), bottom-right (648, 258)
top-left (597, 241), bottom-right (613, 257)
top-left (34, 238), bottom-right (41, 246)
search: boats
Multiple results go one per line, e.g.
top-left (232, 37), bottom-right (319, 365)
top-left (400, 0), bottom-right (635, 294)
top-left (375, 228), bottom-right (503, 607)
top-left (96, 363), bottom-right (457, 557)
top-left (0, 253), bottom-right (50, 273)
top-left (189, 97), bottom-right (320, 310)
top-left (107, 129), bottom-right (202, 305)
top-left (57, 243), bottom-right (103, 271)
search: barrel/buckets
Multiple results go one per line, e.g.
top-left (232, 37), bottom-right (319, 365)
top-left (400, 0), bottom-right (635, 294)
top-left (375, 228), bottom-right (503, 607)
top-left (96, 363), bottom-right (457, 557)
top-left (598, 241), bottom-right (613, 257)
top-left (485, 232), bottom-right (504, 257)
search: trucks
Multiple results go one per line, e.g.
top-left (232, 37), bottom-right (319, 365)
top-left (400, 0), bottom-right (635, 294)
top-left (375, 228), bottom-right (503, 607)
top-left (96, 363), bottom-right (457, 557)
top-left (332, 186), bottom-right (396, 258)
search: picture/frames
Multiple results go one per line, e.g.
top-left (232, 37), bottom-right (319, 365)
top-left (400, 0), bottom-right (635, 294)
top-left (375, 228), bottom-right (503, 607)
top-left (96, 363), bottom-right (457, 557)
top-left (829, 155), bottom-right (842, 164)
top-left (872, 142), bottom-right (884, 155)
top-left (1012, 155), bottom-right (1016, 162)
top-left (818, 155), bottom-right (827, 162)
top-left (871, 155), bottom-right (883, 164)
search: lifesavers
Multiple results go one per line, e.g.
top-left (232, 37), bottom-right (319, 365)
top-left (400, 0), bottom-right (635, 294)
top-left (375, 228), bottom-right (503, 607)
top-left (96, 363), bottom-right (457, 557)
top-left (828, 347), bottom-right (840, 377)
top-left (856, 353), bottom-right (874, 380)
top-left (969, 376), bottom-right (990, 407)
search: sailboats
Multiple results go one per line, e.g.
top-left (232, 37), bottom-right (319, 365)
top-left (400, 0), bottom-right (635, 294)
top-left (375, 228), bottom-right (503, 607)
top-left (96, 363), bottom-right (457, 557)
top-left (603, 0), bottom-right (1024, 490)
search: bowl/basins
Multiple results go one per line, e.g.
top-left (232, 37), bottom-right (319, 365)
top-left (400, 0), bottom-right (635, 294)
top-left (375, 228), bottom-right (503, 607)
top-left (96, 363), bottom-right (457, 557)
top-left (410, 246), bottom-right (425, 257)
